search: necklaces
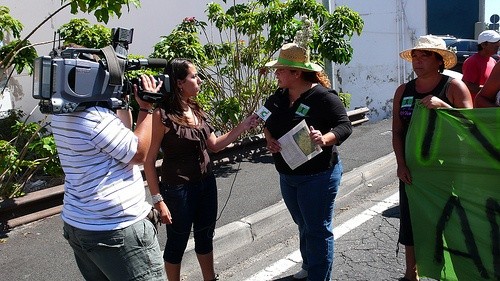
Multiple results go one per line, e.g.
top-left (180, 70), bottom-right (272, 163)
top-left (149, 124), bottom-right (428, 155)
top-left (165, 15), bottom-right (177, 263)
top-left (289, 93), bottom-right (298, 104)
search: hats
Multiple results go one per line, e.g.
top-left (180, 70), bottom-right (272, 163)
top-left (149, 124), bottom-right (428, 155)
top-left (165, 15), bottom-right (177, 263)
top-left (398, 35), bottom-right (457, 70)
top-left (478, 30), bottom-right (500, 44)
top-left (265, 42), bottom-right (323, 72)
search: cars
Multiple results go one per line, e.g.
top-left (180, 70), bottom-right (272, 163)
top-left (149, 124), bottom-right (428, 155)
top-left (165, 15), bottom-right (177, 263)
top-left (446, 38), bottom-right (500, 75)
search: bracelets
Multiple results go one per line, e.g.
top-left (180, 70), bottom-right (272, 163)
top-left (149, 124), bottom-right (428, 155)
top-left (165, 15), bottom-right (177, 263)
top-left (138, 107), bottom-right (153, 114)
top-left (150, 193), bottom-right (164, 205)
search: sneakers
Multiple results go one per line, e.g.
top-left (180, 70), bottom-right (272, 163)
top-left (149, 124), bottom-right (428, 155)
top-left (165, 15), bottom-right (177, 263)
top-left (294, 268), bottom-right (308, 279)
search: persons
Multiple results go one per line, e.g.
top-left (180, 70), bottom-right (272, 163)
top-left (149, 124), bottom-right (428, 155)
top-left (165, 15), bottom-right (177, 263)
top-left (262, 43), bottom-right (352, 281)
top-left (145, 58), bottom-right (265, 281)
top-left (392, 35), bottom-right (473, 281)
top-left (47, 44), bottom-right (170, 281)
top-left (461, 30), bottom-right (500, 104)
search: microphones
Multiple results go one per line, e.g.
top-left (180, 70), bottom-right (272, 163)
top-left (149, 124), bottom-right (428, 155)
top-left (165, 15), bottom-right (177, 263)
top-left (249, 103), bottom-right (272, 130)
top-left (129, 58), bottom-right (167, 68)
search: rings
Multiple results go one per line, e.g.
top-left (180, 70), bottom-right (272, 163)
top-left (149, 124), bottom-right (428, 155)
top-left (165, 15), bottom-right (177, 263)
top-left (269, 146), bottom-right (271, 149)
top-left (401, 177), bottom-right (407, 182)
